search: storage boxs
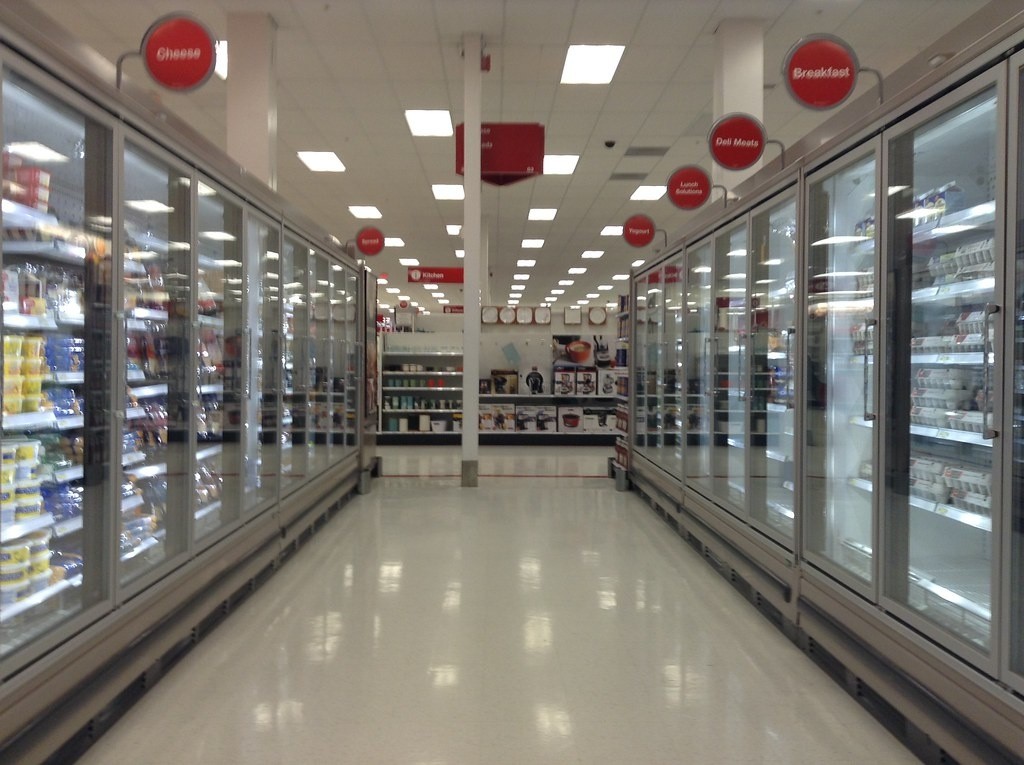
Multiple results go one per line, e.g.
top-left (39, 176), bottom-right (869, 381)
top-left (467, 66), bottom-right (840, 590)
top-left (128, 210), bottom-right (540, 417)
top-left (480, 402), bottom-right (618, 433)
top-left (551, 333), bottom-right (630, 397)
top-left (491, 368), bottom-right (520, 396)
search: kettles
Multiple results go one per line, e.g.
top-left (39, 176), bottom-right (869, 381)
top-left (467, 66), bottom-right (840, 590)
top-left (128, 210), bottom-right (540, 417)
top-left (537, 413), bottom-right (550, 430)
top-left (517, 414), bottom-right (529, 429)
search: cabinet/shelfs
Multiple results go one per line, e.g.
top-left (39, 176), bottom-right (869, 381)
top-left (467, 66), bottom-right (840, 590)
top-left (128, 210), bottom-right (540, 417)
top-left (609, 292), bottom-right (632, 492)
top-left (478, 394), bottom-right (620, 435)
top-left (376, 352), bottom-right (465, 437)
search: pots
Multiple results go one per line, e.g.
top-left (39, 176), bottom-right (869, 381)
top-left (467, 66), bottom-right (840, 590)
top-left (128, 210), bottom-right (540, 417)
top-left (563, 414), bottom-right (580, 426)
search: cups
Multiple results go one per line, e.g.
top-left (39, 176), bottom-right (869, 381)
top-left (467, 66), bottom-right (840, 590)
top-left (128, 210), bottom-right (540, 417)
top-left (395, 379), bottom-right (401, 386)
top-left (388, 380), bottom-right (394, 386)
top-left (417, 365), bottom-right (423, 372)
top-left (410, 364), bottom-right (416, 372)
top-left (428, 380), bottom-right (434, 387)
top-left (420, 380), bottom-right (426, 386)
top-left (402, 364), bottom-right (409, 372)
top-left (410, 380), bottom-right (416, 387)
top-left (565, 342), bottom-right (591, 362)
top-left (413, 400), bottom-right (461, 409)
top-left (438, 380), bottom-right (444, 387)
top-left (403, 379), bottom-right (409, 386)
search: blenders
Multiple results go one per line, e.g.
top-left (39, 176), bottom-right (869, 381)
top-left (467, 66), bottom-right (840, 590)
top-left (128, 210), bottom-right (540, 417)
top-left (560, 374), bottom-right (573, 393)
top-left (603, 374), bottom-right (615, 393)
top-left (583, 373), bottom-right (595, 394)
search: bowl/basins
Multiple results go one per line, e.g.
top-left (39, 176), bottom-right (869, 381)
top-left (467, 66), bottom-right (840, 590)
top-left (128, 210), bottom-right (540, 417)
top-left (446, 367), bottom-right (456, 371)
top-left (426, 366), bottom-right (434, 371)
